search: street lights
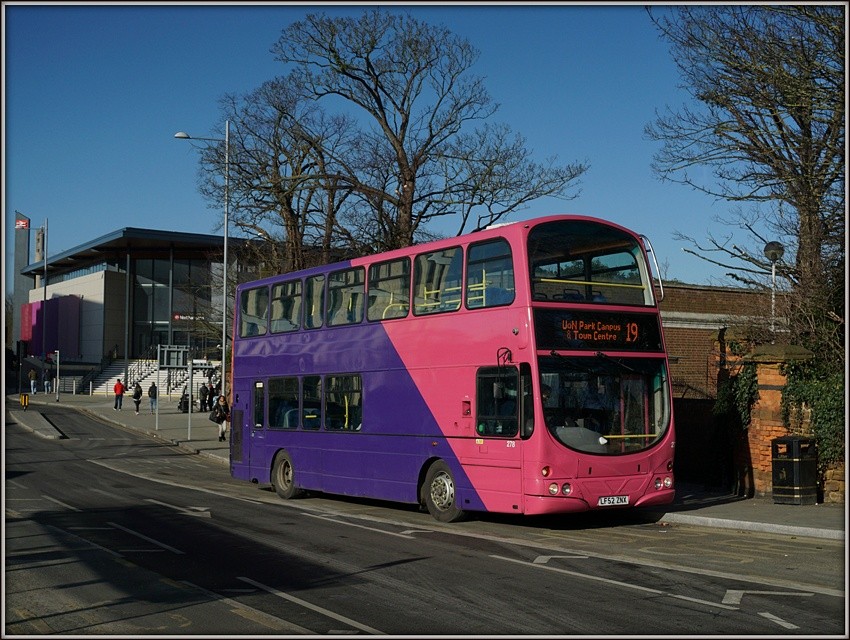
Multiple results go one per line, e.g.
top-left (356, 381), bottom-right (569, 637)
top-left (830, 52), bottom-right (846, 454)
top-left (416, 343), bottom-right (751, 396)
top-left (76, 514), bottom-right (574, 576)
top-left (173, 120), bottom-right (230, 397)
top-left (14, 216), bottom-right (49, 392)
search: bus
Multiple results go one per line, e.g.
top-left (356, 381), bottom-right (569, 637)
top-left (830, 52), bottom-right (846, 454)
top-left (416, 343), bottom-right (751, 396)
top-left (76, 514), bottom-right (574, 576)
top-left (229, 213), bottom-right (677, 523)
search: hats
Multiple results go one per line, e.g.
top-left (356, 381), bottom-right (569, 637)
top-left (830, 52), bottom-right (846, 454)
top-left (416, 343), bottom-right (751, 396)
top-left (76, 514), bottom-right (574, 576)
top-left (208, 384), bottom-right (212, 388)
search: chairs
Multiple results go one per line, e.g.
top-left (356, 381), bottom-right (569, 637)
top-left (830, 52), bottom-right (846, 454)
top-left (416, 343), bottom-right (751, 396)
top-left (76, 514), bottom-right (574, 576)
top-left (259, 399), bottom-right (361, 432)
top-left (439, 285), bottom-right (608, 306)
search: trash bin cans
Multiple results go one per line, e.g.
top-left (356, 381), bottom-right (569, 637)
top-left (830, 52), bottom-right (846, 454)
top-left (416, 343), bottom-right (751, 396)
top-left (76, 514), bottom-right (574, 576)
top-left (182, 394), bottom-right (193, 413)
top-left (769, 436), bottom-right (818, 505)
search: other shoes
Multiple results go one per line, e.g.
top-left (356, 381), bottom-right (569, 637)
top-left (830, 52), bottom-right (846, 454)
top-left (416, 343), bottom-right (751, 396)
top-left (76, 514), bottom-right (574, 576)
top-left (49, 392), bottom-right (52, 394)
top-left (45, 392), bottom-right (47, 394)
top-left (34, 392), bottom-right (36, 394)
top-left (119, 409), bottom-right (121, 411)
top-left (32, 393), bottom-right (34, 394)
top-left (136, 412), bottom-right (139, 415)
top-left (113, 407), bottom-right (116, 411)
top-left (222, 433), bottom-right (225, 440)
top-left (219, 437), bottom-right (222, 442)
top-left (152, 412), bottom-right (153, 414)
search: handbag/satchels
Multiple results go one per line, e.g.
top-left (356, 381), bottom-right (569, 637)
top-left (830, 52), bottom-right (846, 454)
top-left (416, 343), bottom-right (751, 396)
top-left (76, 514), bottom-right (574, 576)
top-left (209, 411), bottom-right (223, 424)
top-left (200, 393), bottom-right (205, 400)
top-left (227, 412), bottom-right (231, 422)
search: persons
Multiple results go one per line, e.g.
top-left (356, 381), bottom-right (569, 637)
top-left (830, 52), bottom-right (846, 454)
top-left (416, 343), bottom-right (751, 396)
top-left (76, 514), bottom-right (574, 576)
top-left (113, 379), bottom-right (125, 411)
top-left (132, 383), bottom-right (143, 415)
top-left (148, 382), bottom-right (159, 415)
top-left (213, 396), bottom-right (229, 442)
top-left (42, 369), bottom-right (52, 395)
top-left (28, 369), bottom-right (38, 395)
top-left (199, 362), bottom-right (215, 411)
